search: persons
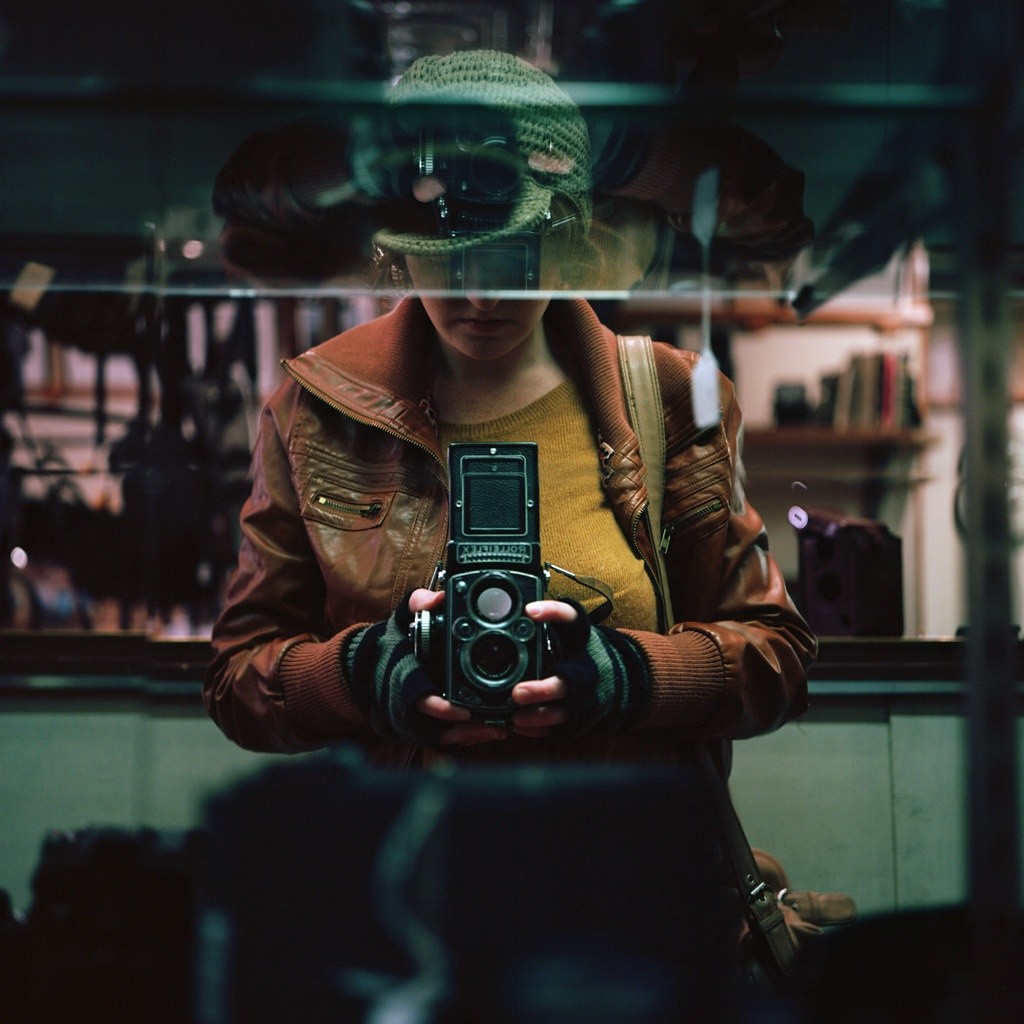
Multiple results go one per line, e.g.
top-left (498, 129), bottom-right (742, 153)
top-left (200, 52), bottom-right (821, 945)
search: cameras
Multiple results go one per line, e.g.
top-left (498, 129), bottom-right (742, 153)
top-left (408, 440), bottom-right (553, 727)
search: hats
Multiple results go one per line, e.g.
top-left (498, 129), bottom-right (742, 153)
top-left (372, 51), bottom-right (593, 257)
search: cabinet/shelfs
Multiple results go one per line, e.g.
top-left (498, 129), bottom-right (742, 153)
top-left (626, 295), bottom-right (935, 449)
